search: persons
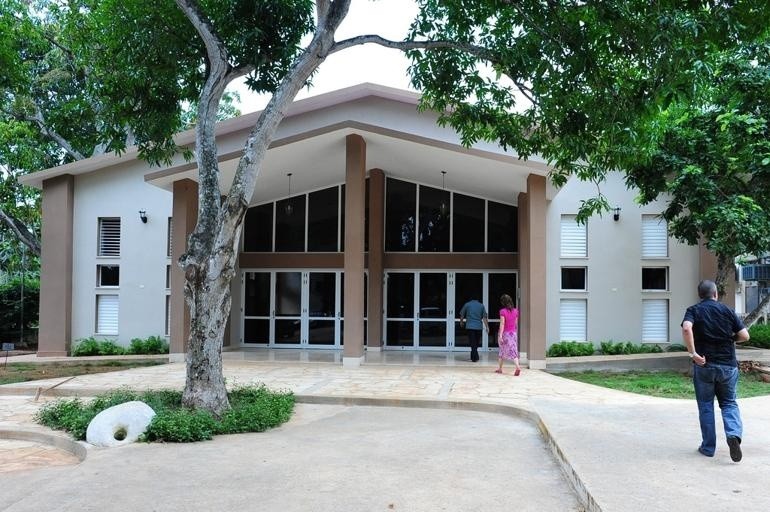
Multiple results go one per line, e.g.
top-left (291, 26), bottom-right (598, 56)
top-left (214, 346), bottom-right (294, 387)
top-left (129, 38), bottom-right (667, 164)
top-left (494, 293), bottom-right (520, 376)
top-left (681, 279), bottom-right (750, 462)
top-left (459, 293), bottom-right (489, 362)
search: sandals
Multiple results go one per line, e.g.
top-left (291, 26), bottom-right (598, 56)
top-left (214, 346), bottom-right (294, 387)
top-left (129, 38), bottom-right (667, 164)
top-left (496, 368), bottom-right (521, 376)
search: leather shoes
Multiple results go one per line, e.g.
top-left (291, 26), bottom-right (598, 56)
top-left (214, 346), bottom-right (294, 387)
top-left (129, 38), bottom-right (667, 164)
top-left (699, 448), bottom-right (714, 456)
top-left (727, 435), bottom-right (742, 462)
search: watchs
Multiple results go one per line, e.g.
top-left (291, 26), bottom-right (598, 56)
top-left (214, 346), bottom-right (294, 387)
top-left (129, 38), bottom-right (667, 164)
top-left (688, 350), bottom-right (697, 359)
